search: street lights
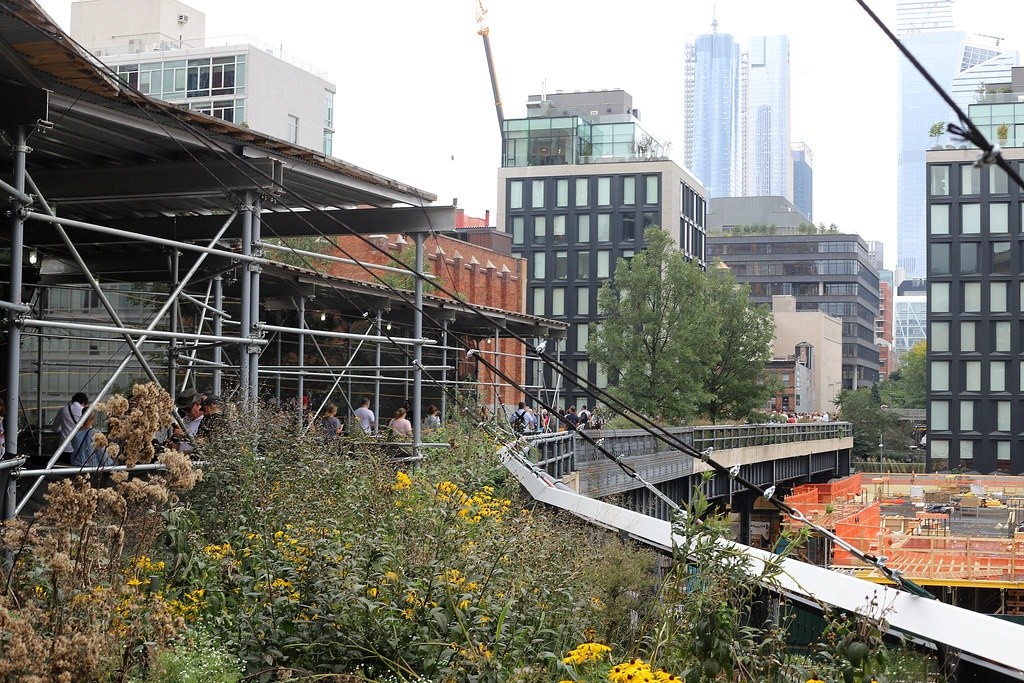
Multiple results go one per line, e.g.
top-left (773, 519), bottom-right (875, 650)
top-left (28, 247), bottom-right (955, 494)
top-left (878, 434), bottom-right (883, 479)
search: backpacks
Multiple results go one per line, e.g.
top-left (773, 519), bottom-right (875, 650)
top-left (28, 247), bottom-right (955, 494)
top-left (581, 411), bottom-right (588, 423)
top-left (514, 411), bottom-right (530, 432)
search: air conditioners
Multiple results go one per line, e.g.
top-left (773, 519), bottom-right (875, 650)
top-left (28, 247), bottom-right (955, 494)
top-left (178, 15), bottom-right (188, 23)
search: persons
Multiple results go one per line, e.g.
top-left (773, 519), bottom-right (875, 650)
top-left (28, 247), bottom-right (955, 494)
top-left (151, 388), bottom-right (230, 463)
top-left (481, 402), bottom-right (604, 436)
top-left (769, 411), bottom-right (838, 423)
top-left (70, 406), bottom-right (119, 466)
top-left (266, 397), bottom-right (344, 440)
top-left (424, 406), bottom-right (440, 428)
top-left (52, 393), bottom-right (93, 465)
top-left (389, 408), bottom-right (413, 474)
top-left (402, 400), bottom-right (413, 428)
top-left (352, 398), bottom-right (374, 436)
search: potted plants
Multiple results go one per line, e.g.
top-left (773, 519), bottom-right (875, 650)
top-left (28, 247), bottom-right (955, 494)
top-left (928, 122), bottom-right (945, 150)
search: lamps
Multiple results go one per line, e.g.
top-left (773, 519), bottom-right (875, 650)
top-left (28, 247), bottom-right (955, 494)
top-left (29, 247), bottom-right (37, 264)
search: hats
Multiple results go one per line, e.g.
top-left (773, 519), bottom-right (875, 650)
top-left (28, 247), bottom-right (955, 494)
top-left (175, 388), bottom-right (202, 408)
top-left (199, 395), bottom-right (223, 411)
top-left (304, 396), bottom-right (308, 403)
